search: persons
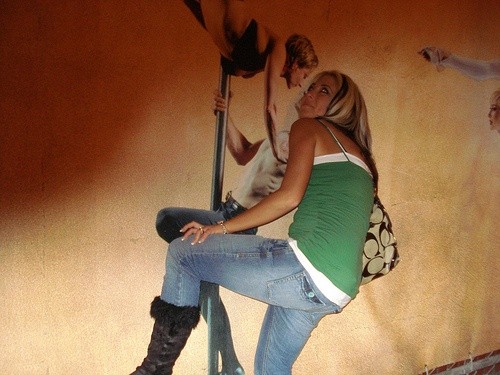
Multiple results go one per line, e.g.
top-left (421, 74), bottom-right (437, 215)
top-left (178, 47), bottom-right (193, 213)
top-left (419, 46), bottom-right (500, 134)
top-left (129, 68), bottom-right (378, 375)
top-left (156, 89), bottom-right (290, 375)
top-left (181, 0), bottom-right (318, 164)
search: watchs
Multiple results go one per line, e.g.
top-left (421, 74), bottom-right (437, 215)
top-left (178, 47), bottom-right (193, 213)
top-left (215, 220), bottom-right (229, 235)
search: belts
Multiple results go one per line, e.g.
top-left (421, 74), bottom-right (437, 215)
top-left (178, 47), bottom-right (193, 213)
top-left (225, 191), bottom-right (245, 214)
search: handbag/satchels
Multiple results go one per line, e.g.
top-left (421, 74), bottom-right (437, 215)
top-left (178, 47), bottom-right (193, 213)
top-left (360, 192), bottom-right (401, 286)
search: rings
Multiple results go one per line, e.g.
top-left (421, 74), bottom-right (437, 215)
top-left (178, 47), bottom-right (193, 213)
top-left (197, 227), bottom-right (204, 236)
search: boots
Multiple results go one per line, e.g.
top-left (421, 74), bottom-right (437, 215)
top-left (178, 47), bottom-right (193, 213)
top-left (128, 295), bottom-right (201, 375)
